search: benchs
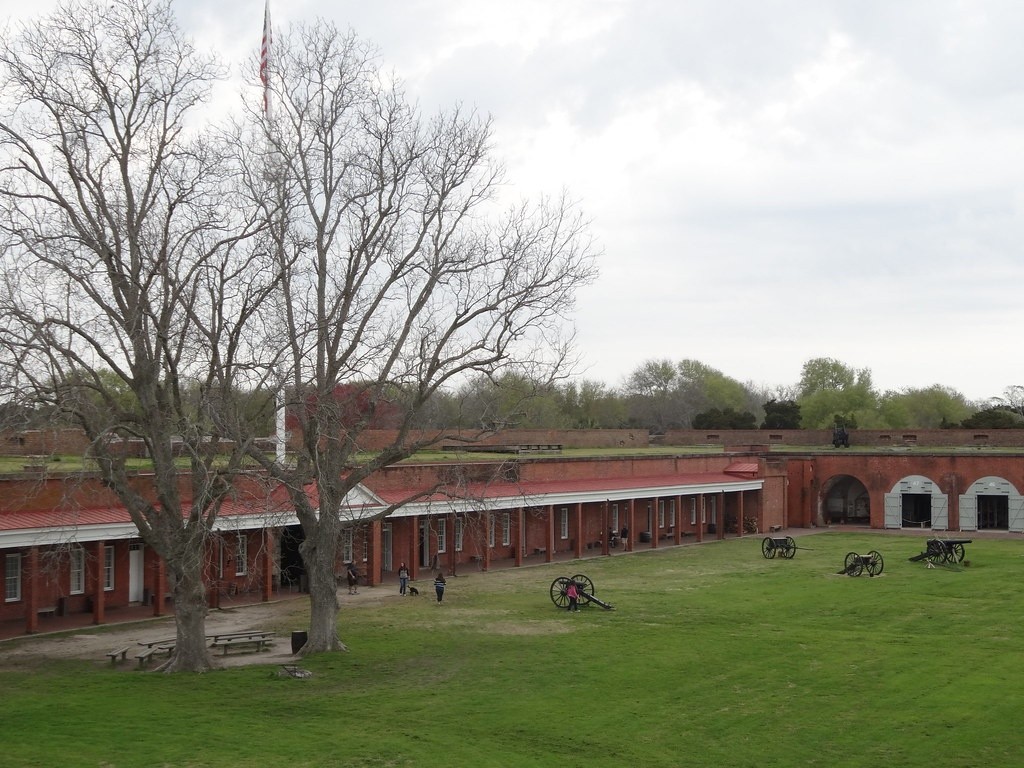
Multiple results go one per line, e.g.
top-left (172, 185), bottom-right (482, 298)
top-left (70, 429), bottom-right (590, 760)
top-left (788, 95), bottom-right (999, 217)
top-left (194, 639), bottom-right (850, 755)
top-left (137, 636), bottom-right (177, 648)
top-left (158, 644), bottom-right (177, 656)
top-left (105, 645), bottom-right (132, 666)
top-left (216, 631), bottom-right (276, 642)
top-left (135, 647), bottom-right (157, 666)
top-left (211, 639), bottom-right (273, 655)
top-left (204, 630), bottom-right (265, 645)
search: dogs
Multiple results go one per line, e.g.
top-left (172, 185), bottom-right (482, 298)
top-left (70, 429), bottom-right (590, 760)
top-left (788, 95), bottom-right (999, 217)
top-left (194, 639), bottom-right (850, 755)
top-left (407, 585), bottom-right (419, 596)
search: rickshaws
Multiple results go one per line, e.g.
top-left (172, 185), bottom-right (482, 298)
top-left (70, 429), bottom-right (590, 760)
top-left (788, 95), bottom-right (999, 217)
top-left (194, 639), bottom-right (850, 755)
top-left (909, 539), bottom-right (973, 562)
top-left (836, 551), bottom-right (884, 577)
top-left (762, 536), bottom-right (796, 559)
top-left (550, 575), bottom-right (612, 609)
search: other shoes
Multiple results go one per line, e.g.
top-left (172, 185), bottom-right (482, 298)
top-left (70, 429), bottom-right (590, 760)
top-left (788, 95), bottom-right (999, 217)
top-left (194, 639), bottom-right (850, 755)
top-left (404, 594), bottom-right (406, 597)
top-left (568, 610), bottom-right (573, 614)
top-left (349, 591), bottom-right (360, 595)
top-left (575, 610), bottom-right (581, 612)
top-left (400, 593), bottom-right (402, 595)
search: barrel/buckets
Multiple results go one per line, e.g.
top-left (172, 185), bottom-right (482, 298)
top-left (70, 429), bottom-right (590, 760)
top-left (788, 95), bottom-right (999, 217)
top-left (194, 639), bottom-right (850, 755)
top-left (642, 531), bottom-right (651, 543)
top-left (710, 523), bottom-right (716, 534)
top-left (291, 631), bottom-right (308, 654)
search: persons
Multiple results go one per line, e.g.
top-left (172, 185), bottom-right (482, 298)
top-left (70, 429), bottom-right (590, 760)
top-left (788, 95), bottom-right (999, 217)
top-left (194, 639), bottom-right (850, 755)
top-left (347, 561), bottom-right (360, 594)
top-left (398, 562), bottom-right (410, 596)
top-left (565, 580), bottom-right (580, 613)
top-left (621, 524), bottom-right (628, 551)
top-left (434, 573), bottom-right (447, 604)
top-left (609, 527), bottom-right (616, 548)
